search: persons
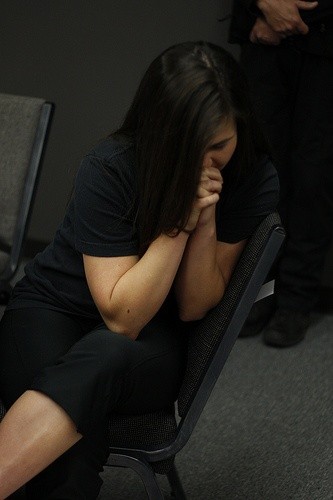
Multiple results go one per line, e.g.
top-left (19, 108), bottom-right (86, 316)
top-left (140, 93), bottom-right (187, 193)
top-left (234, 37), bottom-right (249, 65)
top-left (0, 41), bottom-right (280, 500)
top-left (222, 0), bottom-right (333, 348)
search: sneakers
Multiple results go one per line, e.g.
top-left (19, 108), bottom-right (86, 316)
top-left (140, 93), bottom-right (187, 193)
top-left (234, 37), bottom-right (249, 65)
top-left (236, 298), bottom-right (312, 349)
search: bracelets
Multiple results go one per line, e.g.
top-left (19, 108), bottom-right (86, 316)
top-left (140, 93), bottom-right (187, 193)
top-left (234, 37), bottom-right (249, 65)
top-left (169, 224), bottom-right (195, 234)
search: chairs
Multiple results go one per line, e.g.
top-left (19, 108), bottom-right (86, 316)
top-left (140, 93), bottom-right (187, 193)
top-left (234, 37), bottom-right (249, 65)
top-left (0, 94), bottom-right (55, 305)
top-left (84, 212), bottom-right (286, 500)
